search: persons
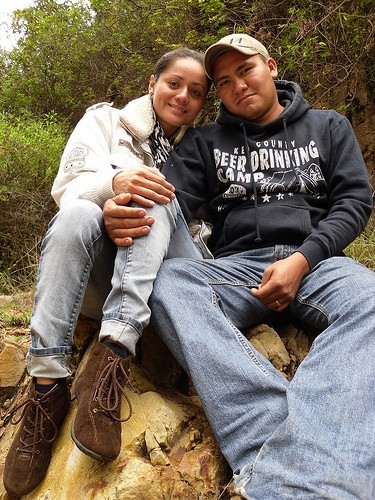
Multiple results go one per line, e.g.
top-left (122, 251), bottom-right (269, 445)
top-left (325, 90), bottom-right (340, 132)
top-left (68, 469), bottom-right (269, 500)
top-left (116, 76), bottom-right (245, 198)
top-left (2, 48), bottom-right (216, 497)
top-left (102, 33), bottom-right (374, 500)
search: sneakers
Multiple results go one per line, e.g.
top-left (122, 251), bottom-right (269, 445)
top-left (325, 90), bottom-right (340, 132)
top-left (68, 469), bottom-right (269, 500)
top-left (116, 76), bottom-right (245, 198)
top-left (0, 377), bottom-right (71, 494)
top-left (72, 344), bottom-right (135, 462)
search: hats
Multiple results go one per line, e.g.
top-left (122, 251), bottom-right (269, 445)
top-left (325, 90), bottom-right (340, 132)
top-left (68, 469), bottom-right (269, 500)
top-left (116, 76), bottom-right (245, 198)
top-left (204, 34), bottom-right (271, 81)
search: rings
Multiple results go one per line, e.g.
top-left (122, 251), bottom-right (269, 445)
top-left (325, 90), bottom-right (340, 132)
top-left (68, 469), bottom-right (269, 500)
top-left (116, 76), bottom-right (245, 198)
top-left (275, 300), bottom-right (282, 307)
